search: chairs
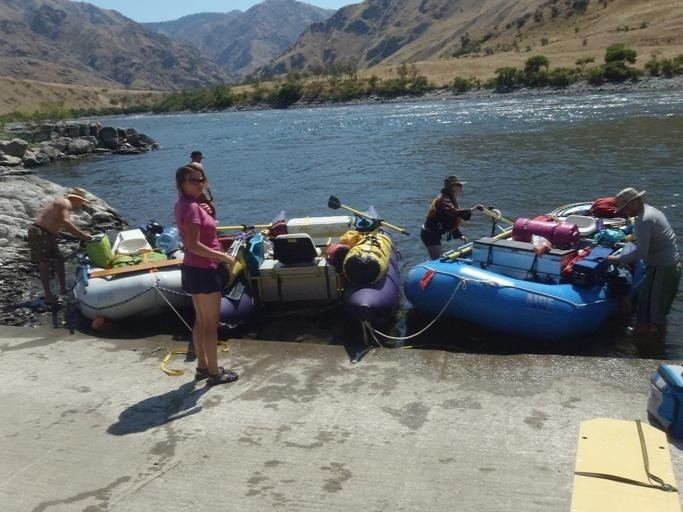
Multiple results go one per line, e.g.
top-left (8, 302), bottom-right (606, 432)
top-left (274, 233), bottom-right (323, 265)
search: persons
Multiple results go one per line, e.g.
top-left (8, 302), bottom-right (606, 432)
top-left (27, 185), bottom-right (96, 310)
top-left (601, 186), bottom-right (682, 345)
top-left (172, 163), bottom-right (237, 387)
top-left (189, 151), bottom-right (216, 223)
top-left (420, 174), bottom-right (485, 262)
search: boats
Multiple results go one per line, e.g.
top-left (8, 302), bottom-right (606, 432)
top-left (404, 200), bottom-right (647, 340)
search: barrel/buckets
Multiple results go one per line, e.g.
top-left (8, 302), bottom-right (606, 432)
top-left (342, 233), bottom-right (393, 286)
top-left (157, 226), bottom-right (180, 254)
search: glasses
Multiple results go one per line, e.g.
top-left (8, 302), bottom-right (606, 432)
top-left (184, 177), bottom-right (207, 186)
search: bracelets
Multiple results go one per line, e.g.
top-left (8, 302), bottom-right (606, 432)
top-left (222, 252), bottom-right (228, 259)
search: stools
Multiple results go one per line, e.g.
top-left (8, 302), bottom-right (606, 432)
top-left (564, 214), bottom-right (596, 237)
top-left (117, 236), bottom-right (151, 254)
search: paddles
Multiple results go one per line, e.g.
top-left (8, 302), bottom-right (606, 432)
top-left (223, 277), bottom-right (247, 301)
top-left (440, 214), bottom-right (552, 263)
top-left (328, 195), bottom-right (410, 236)
top-left (69, 247), bottom-right (89, 287)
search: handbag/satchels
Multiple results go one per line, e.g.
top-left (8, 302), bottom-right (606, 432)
top-left (217, 258), bottom-right (230, 285)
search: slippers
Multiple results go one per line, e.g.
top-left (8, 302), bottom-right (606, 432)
top-left (44, 297), bottom-right (64, 306)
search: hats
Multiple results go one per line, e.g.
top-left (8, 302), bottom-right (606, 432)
top-left (189, 151), bottom-right (203, 159)
top-left (64, 186), bottom-right (89, 203)
top-left (612, 187), bottom-right (647, 215)
top-left (441, 176), bottom-right (468, 193)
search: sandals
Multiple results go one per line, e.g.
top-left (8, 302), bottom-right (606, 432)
top-left (195, 365), bottom-right (223, 380)
top-left (207, 370), bottom-right (238, 386)
